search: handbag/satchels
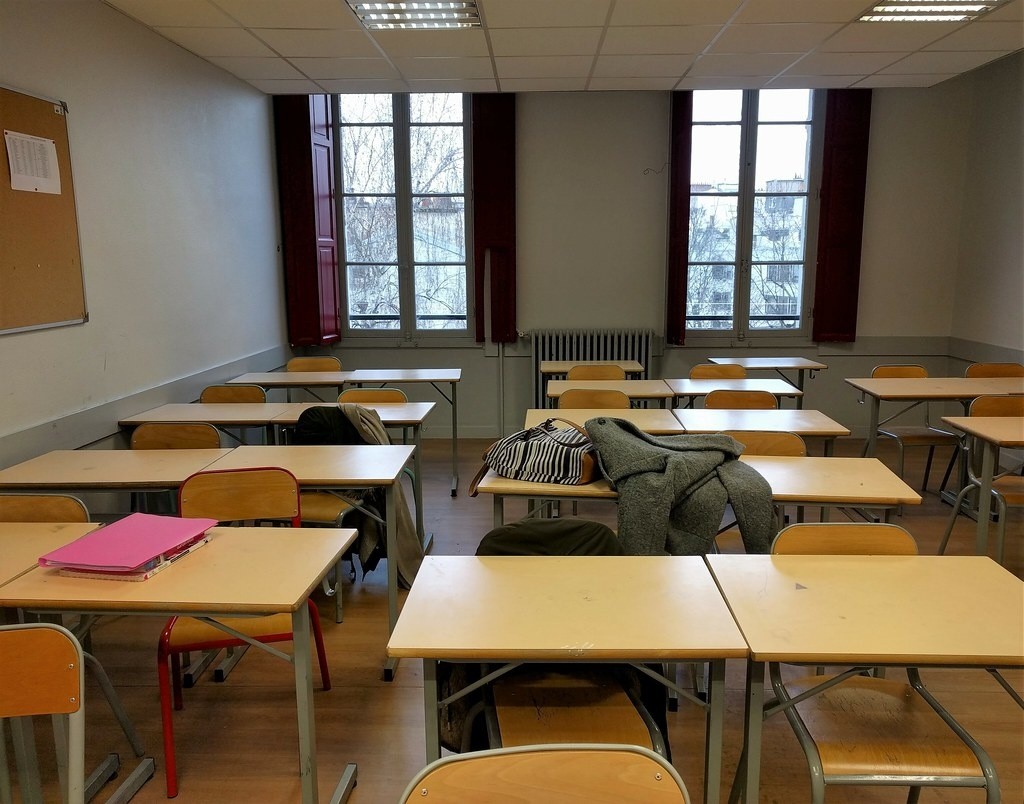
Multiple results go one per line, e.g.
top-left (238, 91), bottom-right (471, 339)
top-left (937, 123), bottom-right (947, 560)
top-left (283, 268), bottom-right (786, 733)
top-left (467, 418), bottom-right (597, 498)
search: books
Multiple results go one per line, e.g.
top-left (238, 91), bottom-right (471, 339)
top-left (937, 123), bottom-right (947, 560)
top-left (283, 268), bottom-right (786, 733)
top-left (60, 533), bottom-right (213, 582)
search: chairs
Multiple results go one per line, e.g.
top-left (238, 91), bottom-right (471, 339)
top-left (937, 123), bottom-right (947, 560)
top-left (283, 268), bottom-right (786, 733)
top-left (0, 354), bottom-right (1024, 804)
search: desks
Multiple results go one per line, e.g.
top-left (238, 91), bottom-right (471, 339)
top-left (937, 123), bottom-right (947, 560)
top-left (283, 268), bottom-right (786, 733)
top-left (271, 402), bottom-right (438, 555)
top-left (948, 377), bottom-right (1024, 521)
top-left (476, 466), bottom-right (619, 528)
top-left (118, 402), bottom-right (303, 446)
top-left (0, 447), bottom-right (234, 686)
top-left (226, 371), bottom-right (353, 404)
top-left (0, 521), bottom-right (120, 804)
top-left (939, 416), bottom-right (1024, 557)
top-left (525, 408), bottom-right (685, 521)
top-left (344, 369), bottom-right (463, 497)
top-left (707, 357), bottom-right (829, 410)
top-left (383, 556), bottom-right (753, 804)
top-left (845, 377), bottom-right (1009, 523)
top-left (672, 408), bottom-right (850, 522)
top-left (704, 554), bottom-right (1024, 804)
top-left (0, 525), bottom-right (357, 804)
top-left (694, 454), bottom-right (923, 702)
top-left (664, 378), bottom-right (804, 409)
top-left (198, 444), bottom-right (418, 680)
top-left (540, 360), bottom-right (645, 374)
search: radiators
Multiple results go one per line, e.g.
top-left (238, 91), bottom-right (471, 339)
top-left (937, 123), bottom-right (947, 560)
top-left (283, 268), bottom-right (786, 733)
top-left (532, 326), bottom-right (655, 409)
